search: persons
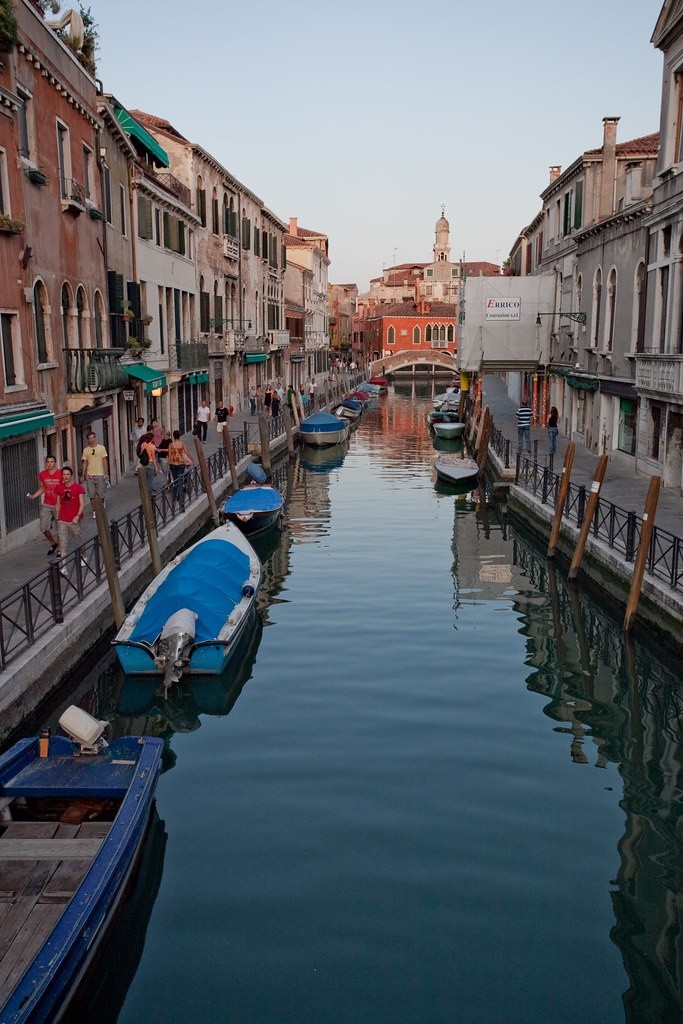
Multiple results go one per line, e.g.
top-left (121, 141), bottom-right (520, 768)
top-left (129, 418), bottom-right (147, 476)
top-left (81, 431), bottom-right (108, 518)
top-left (27, 455), bottom-right (61, 561)
top-left (249, 383), bottom-right (304, 418)
top-left (547, 407), bottom-right (560, 454)
top-left (136, 433), bottom-right (157, 495)
top-left (55, 466), bottom-right (88, 575)
top-left (168, 430), bottom-right (194, 499)
top-left (515, 401), bottom-right (533, 452)
top-left (146, 419), bottom-right (163, 474)
top-left (309, 378), bottom-right (317, 398)
top-left (215, 400), bottom-right (229, 444)
top-left (195, 401), bottom-right (209, 444)
top-left (156, 430), bottom-right (172, 477)
top-left (332, 359), bottom-right (357, 374)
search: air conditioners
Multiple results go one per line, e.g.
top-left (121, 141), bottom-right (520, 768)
top-left (85, 363), bottom-right (112, 393)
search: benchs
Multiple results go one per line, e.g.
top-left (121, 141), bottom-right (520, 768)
top-left (0, 838), bottom-right (105, 861)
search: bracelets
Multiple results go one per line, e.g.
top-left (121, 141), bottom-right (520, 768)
top-left (77, 514), bottom-right (80, 517)
top-left (82, 474), bottom-right (85, 475)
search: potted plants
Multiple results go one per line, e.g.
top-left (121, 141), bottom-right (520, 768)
top-left (123, 311), bottom-right (134, 322)
top-left (143, 314), bottom-right (153, 326)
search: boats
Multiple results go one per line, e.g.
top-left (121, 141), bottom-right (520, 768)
top-left (330, 397), bottom-right (362, 419)
top-left (432, 377), bottom-right (461, 413)
top-left (349, 375), bottom-right (388, 404)
top-left (219, 479), bottom-right (284, 538)
top-left (0, 704), bottom-right (167, 1024)
top-left (434, 478), bottom-right (479, 496)
top-left (434, 449), bottom-right (479, 485)
top-left (110, 520), bottom-right (262, 674)
top-left (298, 408), bottom-right (350, 447)
top-left (431, 416), bottom-right (465, 440)
top-left (432, 436), bottom-right (466, 454)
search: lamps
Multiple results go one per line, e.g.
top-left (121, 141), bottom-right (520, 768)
top-left (535, 309), bottom-right (587, 329)
top-left (210, 318), bottom-right (253, 330)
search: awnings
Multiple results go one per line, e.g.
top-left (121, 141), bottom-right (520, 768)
top-left (124, 365), bottom-right (166, 392)
top-left (114, 109), bottom-right (169, 168)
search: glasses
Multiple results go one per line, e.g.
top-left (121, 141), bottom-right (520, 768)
top-left (92, 449), bottom-right (96, 455)
top-left (65, 490), bottom-right (70, 501)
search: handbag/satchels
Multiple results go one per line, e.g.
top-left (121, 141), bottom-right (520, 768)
top-left (182, 444), bottom-right (194, 466)
top-left (192, 423), bottom-right (198, 435)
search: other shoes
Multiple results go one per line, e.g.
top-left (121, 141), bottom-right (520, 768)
top-left (204, 440), bottom-right (206, 444)
top-left (93, 509), bottom-right (97, 519)
top-left (159, 470), bottom-right (162, 473)
top-left (60, 568), bottom-right (69, 577)
top-left (47, 542), bottom-right (58, 557)
top-left (81, 557), bottom-right (89, 567)
top-left (134, 471), bottom-right (138, 476)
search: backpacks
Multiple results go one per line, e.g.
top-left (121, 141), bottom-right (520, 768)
top-left (140, 444), bottom-right (151, 466)
